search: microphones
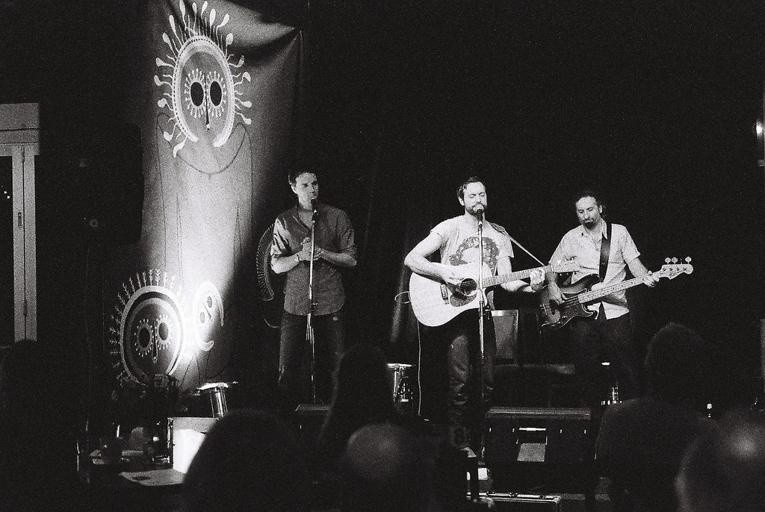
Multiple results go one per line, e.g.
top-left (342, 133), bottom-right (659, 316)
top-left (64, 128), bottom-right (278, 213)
top-left (310, 196), bottom-right (318, 216)
top-left (475, 204), bottom-right (484, 228)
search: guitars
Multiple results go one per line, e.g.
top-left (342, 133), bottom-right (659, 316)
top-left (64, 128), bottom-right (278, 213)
top-left (408, 260), bottom-right (579, 328)
top-left (533, 255), bottom-right (694, 330)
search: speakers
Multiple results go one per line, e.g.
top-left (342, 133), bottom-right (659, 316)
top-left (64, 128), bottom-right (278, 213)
top-left (485, 406), bottom-right (595, 494)
top-left (295, 402), bottom-right (332, 454)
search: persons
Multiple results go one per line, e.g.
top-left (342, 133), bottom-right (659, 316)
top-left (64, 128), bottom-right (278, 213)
top-left (547, 190), bottom-right (659, 403)
top-left (404, 176), bottom-right (548, 464)
top-left (270, 166), bottom-right (358, 420)
